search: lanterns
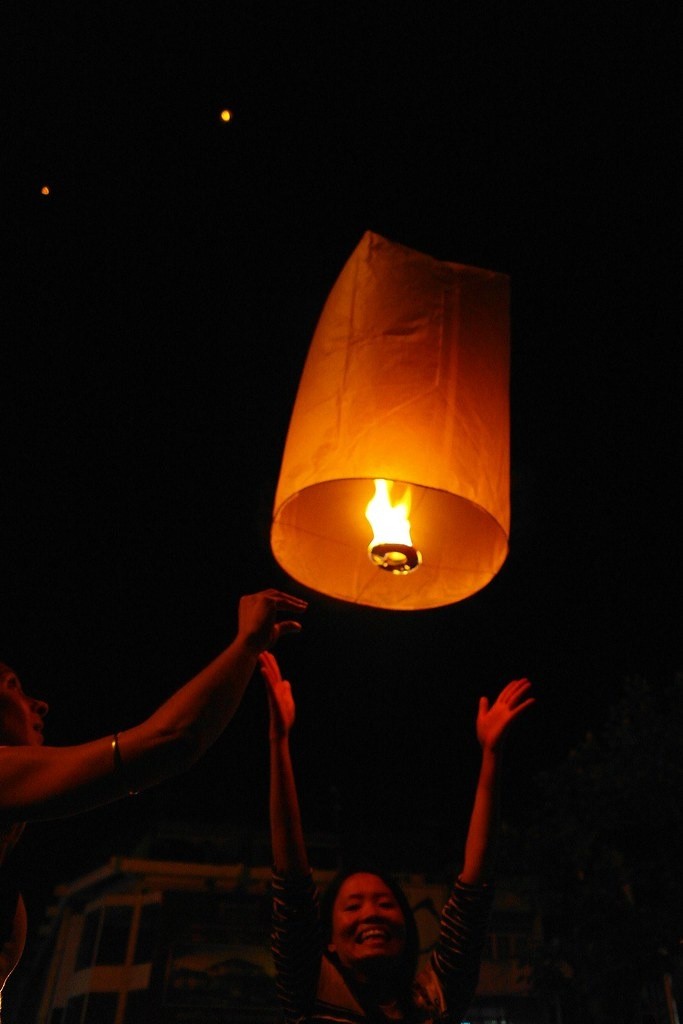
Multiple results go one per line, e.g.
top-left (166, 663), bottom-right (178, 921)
top-left (269, 231), bottom-right (512, 613)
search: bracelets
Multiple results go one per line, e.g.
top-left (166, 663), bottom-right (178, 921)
top-left (110, 730), bottom-right (141, 796)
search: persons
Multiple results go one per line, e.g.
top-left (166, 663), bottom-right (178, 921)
top-left (257, 651), bottom-right (535, 1024)
top-left (0, 588), bottom-right (307, 1000)
